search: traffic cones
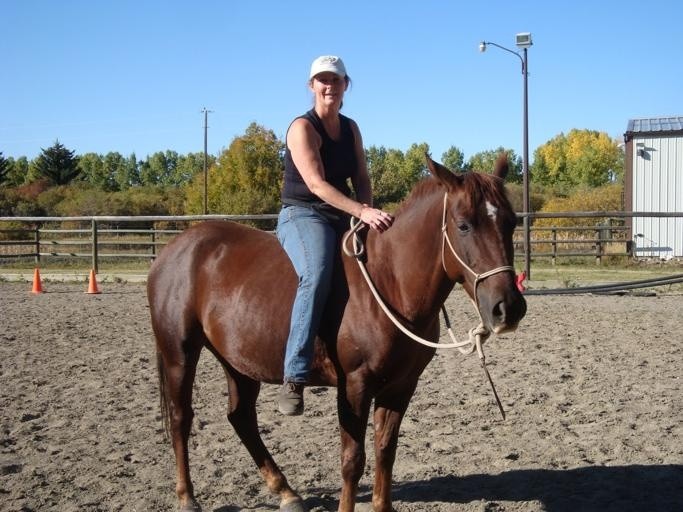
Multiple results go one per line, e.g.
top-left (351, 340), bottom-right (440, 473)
top-left (83, 269), bottom-right (103, 294)
top-left (26, 268), bottom-right (47, 293)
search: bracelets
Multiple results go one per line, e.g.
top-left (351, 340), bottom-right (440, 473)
top-left (358, 205), bottom-right (366, 217)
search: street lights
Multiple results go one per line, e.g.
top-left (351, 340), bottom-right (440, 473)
top-left (479, 31), bottom-right (533, 280)
top-left (201, 106), bottom-right (212, 214)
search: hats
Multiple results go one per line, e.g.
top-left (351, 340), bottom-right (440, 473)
top-left (310, 55), bottom-right (346, 81)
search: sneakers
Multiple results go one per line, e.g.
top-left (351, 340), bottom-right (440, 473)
top-left (279, 383), bottom-right (303, 416)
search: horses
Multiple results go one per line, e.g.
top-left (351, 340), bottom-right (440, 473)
top-left (145, 150), bottom-right (528, 512)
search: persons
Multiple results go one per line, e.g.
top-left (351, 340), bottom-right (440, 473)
top-left (274, 53), bottom-right (395, 416)
